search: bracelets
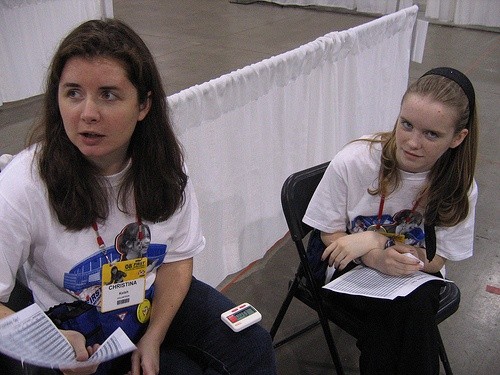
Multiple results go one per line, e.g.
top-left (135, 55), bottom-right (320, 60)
top-left (384, 237), bottom-right (396, 250)
top-left (353, 256), bottom-right (368, 268)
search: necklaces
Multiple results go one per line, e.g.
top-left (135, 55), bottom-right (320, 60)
top-left (372, 160), bottom-right (433, 243)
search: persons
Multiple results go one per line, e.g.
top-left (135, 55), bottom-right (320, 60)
top-left (0, 13), bottom-right (277, 375)
top-left (300, 67), bottom-right (479, 375)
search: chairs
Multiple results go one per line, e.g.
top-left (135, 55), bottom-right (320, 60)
top-left (269, 160), bottom-right (461, 375)
